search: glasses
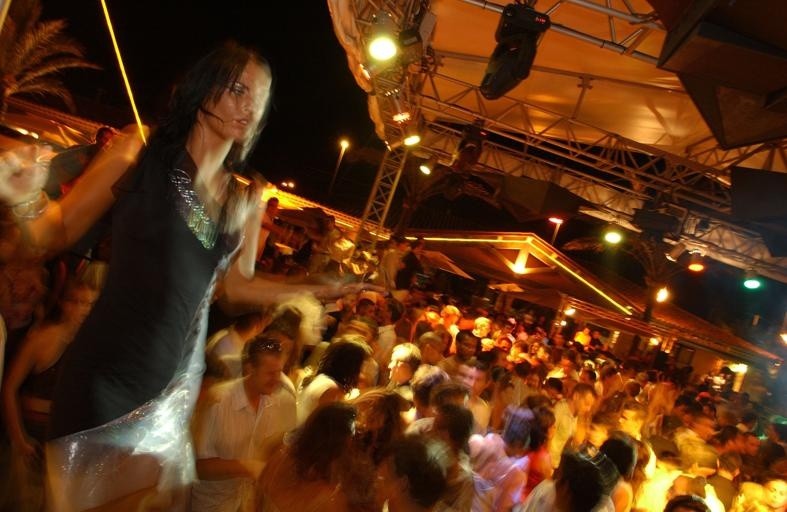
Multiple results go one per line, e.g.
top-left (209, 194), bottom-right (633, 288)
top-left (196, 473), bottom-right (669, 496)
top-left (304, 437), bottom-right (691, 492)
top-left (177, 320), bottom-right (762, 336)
top-left (260, 341), bottom-right (283, 353)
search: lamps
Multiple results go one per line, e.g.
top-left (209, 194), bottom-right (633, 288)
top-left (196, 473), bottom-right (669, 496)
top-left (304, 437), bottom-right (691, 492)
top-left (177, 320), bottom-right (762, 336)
top-left (660, 239), bottom-right (763, 292)
top-left (418, 154), bottom-right (439, 174)
top-left (366, 10), bottom-right (421, 147)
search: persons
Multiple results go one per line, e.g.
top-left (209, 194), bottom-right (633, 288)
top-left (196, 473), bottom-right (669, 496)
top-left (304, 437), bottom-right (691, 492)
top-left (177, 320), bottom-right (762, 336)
top-left (0, 121), bottom-right (786, 511)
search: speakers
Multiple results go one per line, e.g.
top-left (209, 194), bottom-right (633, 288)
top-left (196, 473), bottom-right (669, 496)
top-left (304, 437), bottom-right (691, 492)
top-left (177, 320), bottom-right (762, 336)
top-left (432, 266), bottom-right (475, 306)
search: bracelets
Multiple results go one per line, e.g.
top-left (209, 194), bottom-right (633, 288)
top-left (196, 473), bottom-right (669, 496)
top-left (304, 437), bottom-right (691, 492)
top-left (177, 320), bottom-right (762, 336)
top-left (0, 37), bottom-right (275, 512)
